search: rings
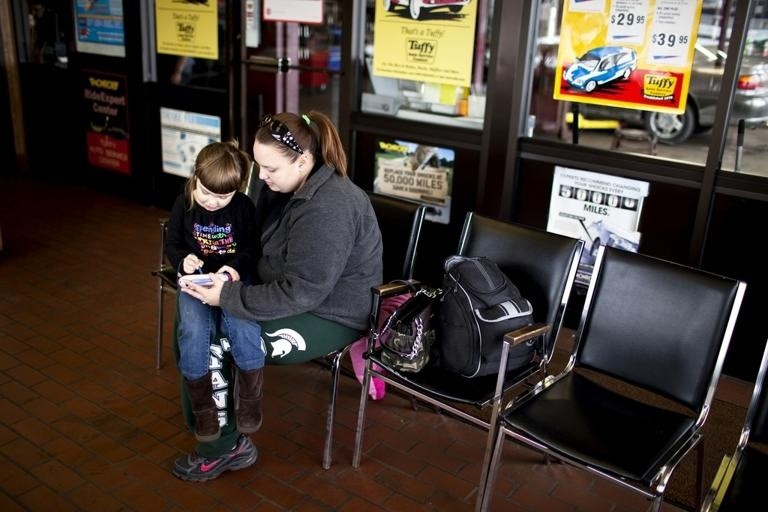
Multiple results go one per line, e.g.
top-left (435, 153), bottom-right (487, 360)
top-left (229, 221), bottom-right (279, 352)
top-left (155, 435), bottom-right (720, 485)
top-left (202, 299), bottom-right (208, 304)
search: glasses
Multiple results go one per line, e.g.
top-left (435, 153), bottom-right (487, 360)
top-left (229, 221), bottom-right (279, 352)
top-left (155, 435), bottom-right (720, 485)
top-left (259, 114), bottom-right (305, 156)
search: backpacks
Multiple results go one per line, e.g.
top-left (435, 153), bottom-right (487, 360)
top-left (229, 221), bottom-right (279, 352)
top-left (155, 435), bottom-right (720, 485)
top-left (438, 251), bottom-right (538, 382)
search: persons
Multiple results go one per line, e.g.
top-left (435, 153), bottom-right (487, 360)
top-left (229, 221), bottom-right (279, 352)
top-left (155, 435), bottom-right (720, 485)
top-left (30, 0), bottom-right (70, 62)
top-left (164, 139), bottom-right (265, 443)
top-left (172, 110), bottom-right (384, 482)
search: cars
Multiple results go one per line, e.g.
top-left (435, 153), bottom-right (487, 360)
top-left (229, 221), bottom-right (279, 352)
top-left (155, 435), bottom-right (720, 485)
top-left (324, 24), bottom-right (343, 72)
top-left (536, 32), bottom-right (768, 146)
top-left (560, 49), bottom-right (635, 93)
top-left (382, 0), bottom-right (472, 21)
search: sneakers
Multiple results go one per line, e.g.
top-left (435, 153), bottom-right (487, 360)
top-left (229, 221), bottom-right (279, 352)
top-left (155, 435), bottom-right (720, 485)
top-left (169, 432), bottom-right (259, 483)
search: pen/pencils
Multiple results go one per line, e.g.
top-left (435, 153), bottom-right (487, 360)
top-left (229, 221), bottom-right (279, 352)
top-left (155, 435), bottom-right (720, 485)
top-left (198, 266), bottom-right (202, 275)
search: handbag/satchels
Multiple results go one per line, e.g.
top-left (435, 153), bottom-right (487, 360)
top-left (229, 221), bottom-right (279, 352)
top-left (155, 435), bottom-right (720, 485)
top-left (369, 276), bottom-right (444, 375)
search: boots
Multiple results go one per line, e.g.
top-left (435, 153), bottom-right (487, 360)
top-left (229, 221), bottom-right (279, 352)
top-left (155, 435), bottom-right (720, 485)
top-left (233, 364), bottom-right (265, 435)
top-left (182, 368), bottom-right (222, 443)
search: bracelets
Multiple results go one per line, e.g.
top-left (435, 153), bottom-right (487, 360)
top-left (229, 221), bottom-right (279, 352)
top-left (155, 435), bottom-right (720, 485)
top-left (226, 273), bottom-right (232, 282)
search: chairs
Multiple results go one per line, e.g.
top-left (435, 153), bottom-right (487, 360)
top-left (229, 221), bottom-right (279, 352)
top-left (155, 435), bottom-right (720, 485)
top-left (233, 190), bottom-right (430, 470)
top-left (480, 244), bottom-right (748, 512)
top-left (352, 210), bottom-right (585, 512)
top-left (700, 339), bottom-right (768, 512)
top-left (150, 161), bottom-right (266, 371)
